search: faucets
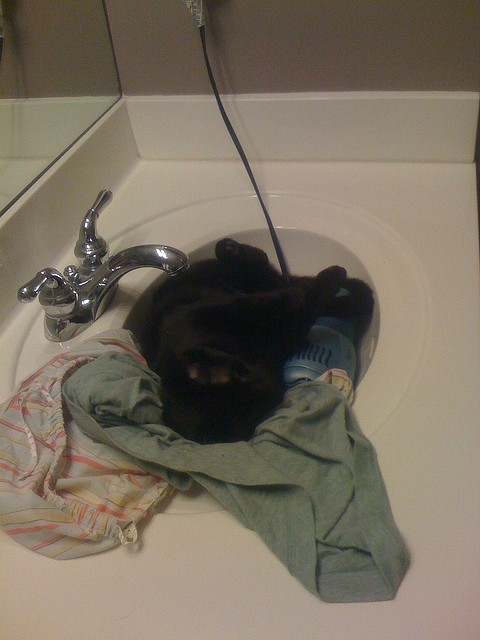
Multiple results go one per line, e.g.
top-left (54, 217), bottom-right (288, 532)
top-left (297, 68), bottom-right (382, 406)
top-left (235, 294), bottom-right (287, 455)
top-left (82, 244), bottom-right (191, 333)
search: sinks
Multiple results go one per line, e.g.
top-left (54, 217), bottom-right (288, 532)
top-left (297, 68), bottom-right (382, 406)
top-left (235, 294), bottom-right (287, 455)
top-left (12, 194), bottom-right (431, 519)
